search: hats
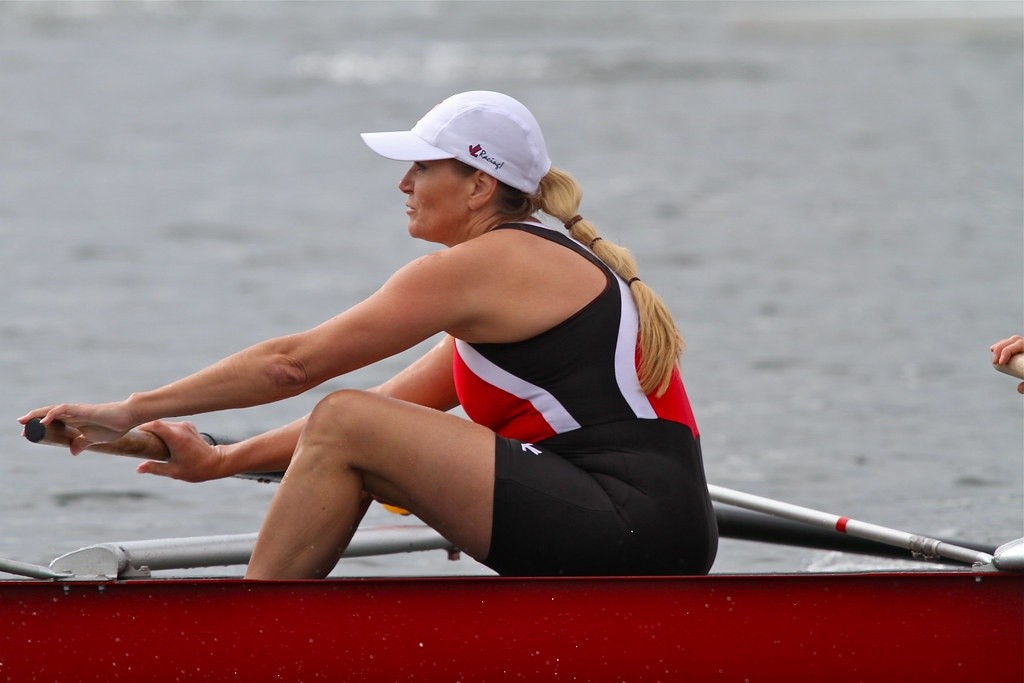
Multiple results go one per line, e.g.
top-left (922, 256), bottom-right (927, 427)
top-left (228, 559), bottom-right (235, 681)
top-left (360, 91), bottom-right (551, 195)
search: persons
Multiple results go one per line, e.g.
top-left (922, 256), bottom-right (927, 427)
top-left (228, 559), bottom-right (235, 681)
top-left (16, 92), bottom-right (719, 580)
top-left (991, 335), bottom-right (1024, 392)
